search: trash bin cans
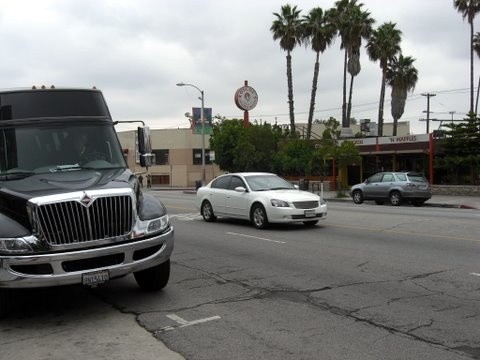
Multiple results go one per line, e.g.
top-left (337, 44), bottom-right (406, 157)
top-left (196, 180), bottom-right (201, 191)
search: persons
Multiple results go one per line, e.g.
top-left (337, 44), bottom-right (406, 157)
top-left (138, 173), bottom-right (144, 188)
top-left (146, 174), bottom-right (152, 189)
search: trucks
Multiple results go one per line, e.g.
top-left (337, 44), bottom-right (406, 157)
top-left (0, 85), bottom-right (174, 299)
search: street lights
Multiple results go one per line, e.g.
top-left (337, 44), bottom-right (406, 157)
top-left (176, 83), bottom-right (205, 185)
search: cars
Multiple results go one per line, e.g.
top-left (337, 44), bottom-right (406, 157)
top-left (196, 172), bottom-right (327, 229)
top-left (350, 172), bottom-right (432, 207)
